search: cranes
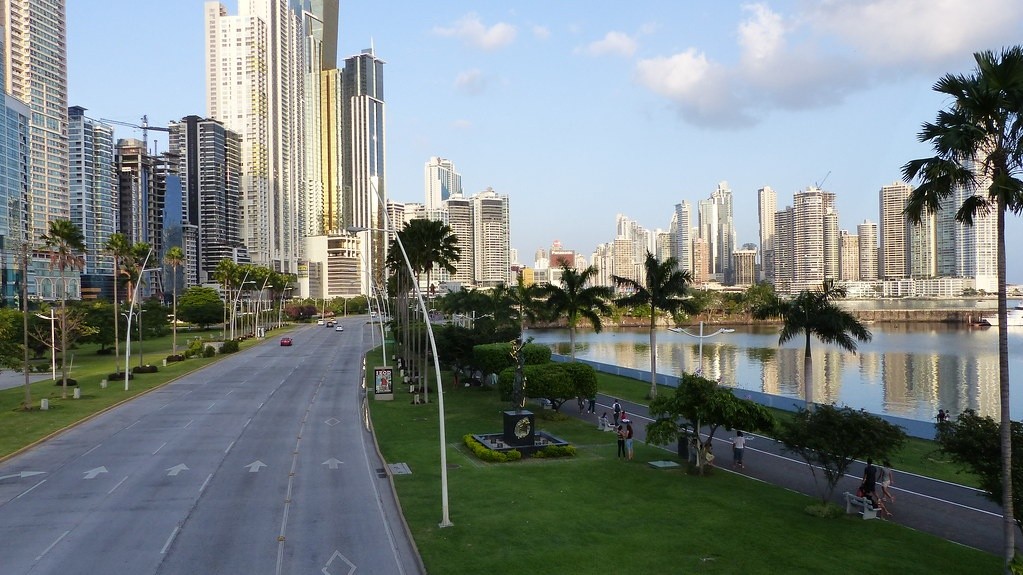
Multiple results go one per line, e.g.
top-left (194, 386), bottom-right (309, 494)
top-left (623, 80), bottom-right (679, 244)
top-left (100, 115), bottom-right (169, 155)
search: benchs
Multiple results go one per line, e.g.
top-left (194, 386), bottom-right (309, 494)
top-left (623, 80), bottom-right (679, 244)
top-left (843, 492), bottom-right (882, 520)
top-left (687, 447), bottom-right (708, 466)
top-left (597, 417), bottom-right (613, 432)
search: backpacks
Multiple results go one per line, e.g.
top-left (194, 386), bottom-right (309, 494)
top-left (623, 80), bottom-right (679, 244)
top-left (615, 404), bottom-right (620, 412)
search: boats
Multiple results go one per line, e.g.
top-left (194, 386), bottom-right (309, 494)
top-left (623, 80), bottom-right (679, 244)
top-left (984, 306), bottom-right (1023, 325)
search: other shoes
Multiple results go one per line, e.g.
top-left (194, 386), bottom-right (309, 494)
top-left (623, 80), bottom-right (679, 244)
top-left (593, 412), bottom-right (596, 414)
top-left (588, 411), bottom-right (589, 414)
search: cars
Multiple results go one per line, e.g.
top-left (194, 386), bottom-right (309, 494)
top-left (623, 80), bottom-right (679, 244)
top-left (326, 318), bottom-right (338, 327)
top-left (318, 319), bottom-right (325, 326)
top-left (281, 337), bottom-right (293, 346)
top-left (336, 326), bottom-right (343, 331)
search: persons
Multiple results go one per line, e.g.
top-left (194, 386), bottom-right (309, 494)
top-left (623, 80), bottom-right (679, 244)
top-left (601, 399), bottom-right (628, 428)
top-left (617, 424), bottom-right (633, 461)
top-left (453, 373), bottom-right (459, 389)
top-left (733, 431), bottom-right (745, 469)
top-left (587, 396), bottom-right (596, 414)
top-left (860, 458), bottom-right (894, 522)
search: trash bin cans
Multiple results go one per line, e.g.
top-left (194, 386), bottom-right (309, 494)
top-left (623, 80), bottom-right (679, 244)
top-left (678, 432), bottom-right (688, 458)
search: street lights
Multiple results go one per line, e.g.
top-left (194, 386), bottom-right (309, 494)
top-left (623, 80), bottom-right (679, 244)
top-left (455, 311), bottom-right (492, 329)
top-left (35, 308), bottom-right (58, 381)
top-left (131, 310), bottom-right (147, 342)
top-left (346, 177), bottom-right (453, 528)
top-left (667, 321), bottom-right (736, 466)
top-left (255, 273), bottom-right (273, 338)
top-left (231, 269), bottom-right (256, 341)
top-left (121, 248), bottom-right (163, 391)
top-left (279, 281), bottom-right (292, 328)
